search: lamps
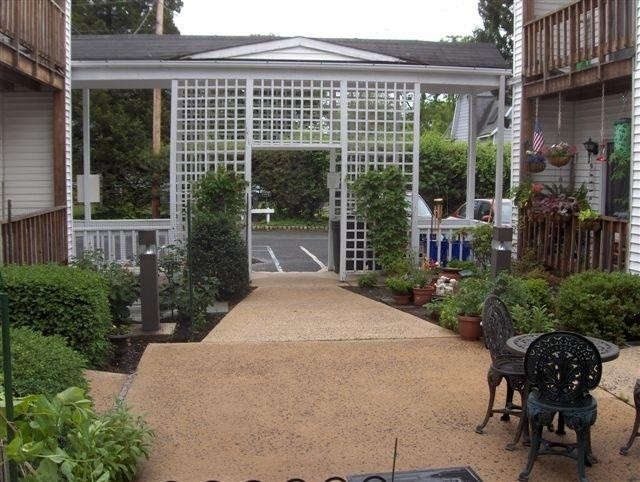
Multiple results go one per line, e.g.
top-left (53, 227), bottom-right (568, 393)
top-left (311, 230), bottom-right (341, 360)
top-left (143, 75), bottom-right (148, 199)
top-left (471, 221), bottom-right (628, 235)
top-left (582, 135), bottom-right (599, 155)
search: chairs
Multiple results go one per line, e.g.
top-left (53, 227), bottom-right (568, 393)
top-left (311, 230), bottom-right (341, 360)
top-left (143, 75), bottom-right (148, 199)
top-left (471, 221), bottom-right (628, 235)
top-left (476, 294), bottom-right (621, 482)
top-left (620, 376), bottom-right (639, 455)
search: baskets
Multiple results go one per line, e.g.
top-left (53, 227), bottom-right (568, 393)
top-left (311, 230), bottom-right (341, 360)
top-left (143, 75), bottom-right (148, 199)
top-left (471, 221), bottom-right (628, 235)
top-left (523, 162), bottom-right (547, 174)
top-left (547, 155), bottom-right (572, 167)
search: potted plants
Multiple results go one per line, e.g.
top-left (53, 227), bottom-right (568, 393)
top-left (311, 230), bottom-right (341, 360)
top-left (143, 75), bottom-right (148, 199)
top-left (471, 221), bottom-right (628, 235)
top-left (525, 137), bottom-right (570, 173)
top-left (384, 272), bottom-right (434, 306)
top-left (93, 262), bottom-right (140, 357)
top-left (450, 274), bottom-right (491, 340)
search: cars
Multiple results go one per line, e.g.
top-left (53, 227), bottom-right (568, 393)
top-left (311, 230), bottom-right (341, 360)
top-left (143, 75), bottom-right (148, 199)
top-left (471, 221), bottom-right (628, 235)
top-left (406, 191), bottom-right (439, 226)
top-left (442, 198), bottom-right (512, 227)
